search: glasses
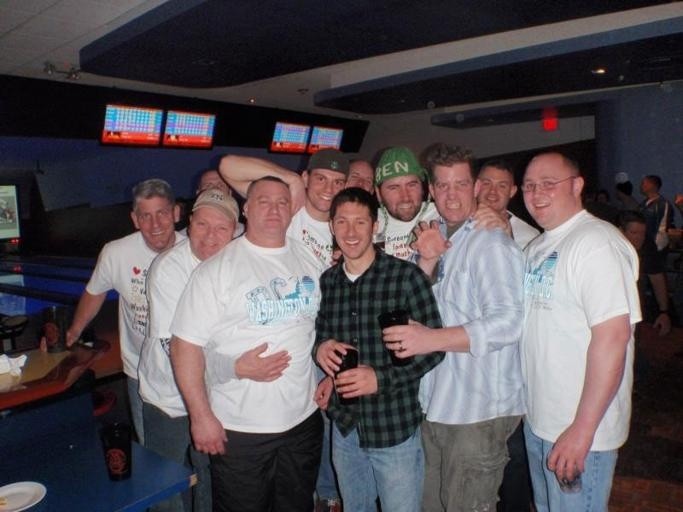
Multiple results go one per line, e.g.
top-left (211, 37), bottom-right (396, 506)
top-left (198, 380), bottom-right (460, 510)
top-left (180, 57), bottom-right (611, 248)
top-left (520, 177), bottom-right (577, 193)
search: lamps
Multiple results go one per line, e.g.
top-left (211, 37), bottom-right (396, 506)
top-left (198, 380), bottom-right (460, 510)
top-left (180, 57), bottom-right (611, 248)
top-left (542, 117), bottom-right (558, 131)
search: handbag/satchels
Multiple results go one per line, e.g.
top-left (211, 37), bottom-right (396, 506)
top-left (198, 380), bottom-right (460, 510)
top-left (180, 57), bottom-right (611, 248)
top-left (653, 222), bottom-right (670, 252)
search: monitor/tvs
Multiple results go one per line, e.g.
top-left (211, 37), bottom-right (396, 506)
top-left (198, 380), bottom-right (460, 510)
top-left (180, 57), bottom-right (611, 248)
top-left (161, 109), bottom-right (219, 148)
top-left (268, 121), bottom-right (311, 153)
top-left (101, 103), bottom-right (164, 146)
top-left (307, 125), bottom-right (345, 154)
top-left (0, 185), bottom-right (21, 239)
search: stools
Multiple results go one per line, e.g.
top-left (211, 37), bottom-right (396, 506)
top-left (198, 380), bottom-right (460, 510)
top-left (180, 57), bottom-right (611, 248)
top-left (92, 389), bottom-right (118, 417)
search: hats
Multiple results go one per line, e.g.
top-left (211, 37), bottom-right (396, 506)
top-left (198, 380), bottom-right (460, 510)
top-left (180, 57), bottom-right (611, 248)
top-left (307, 147), bottom-right (350, 176)
top-left (372, 146), bottom-right (426, 184)
top-left (188, 189), bottom-right (241, 222)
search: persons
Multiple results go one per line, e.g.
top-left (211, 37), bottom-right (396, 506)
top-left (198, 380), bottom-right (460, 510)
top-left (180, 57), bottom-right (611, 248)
top-left (64, 145), bottom-right (683, 512)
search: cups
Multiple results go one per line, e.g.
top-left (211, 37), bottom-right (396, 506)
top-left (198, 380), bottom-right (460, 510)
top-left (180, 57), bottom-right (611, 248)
top-left (98, 425), bottom-right (133, 482)
top-left (39, 305), bottom-right (70, 353)
top-left (378, 308), bottom-right (417, 368)
top-left (329, 349), bottom-right (358, 406)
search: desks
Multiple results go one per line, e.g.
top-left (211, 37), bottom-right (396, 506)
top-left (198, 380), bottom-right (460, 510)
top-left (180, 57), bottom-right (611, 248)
top-left (0, 274), bottom-right (118, 302)
top-left (0, 292), bottom-right (64, 354)
top-left (5, 262), bottom-right (92, 280)
top-left (1, 327), bottom-right (124, 411)
top-left (1, 395), bottom-right (197, 511)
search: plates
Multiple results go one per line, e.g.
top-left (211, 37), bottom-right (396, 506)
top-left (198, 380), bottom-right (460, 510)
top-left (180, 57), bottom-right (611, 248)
top-left (0, 481), bottom-right (47, 511)
